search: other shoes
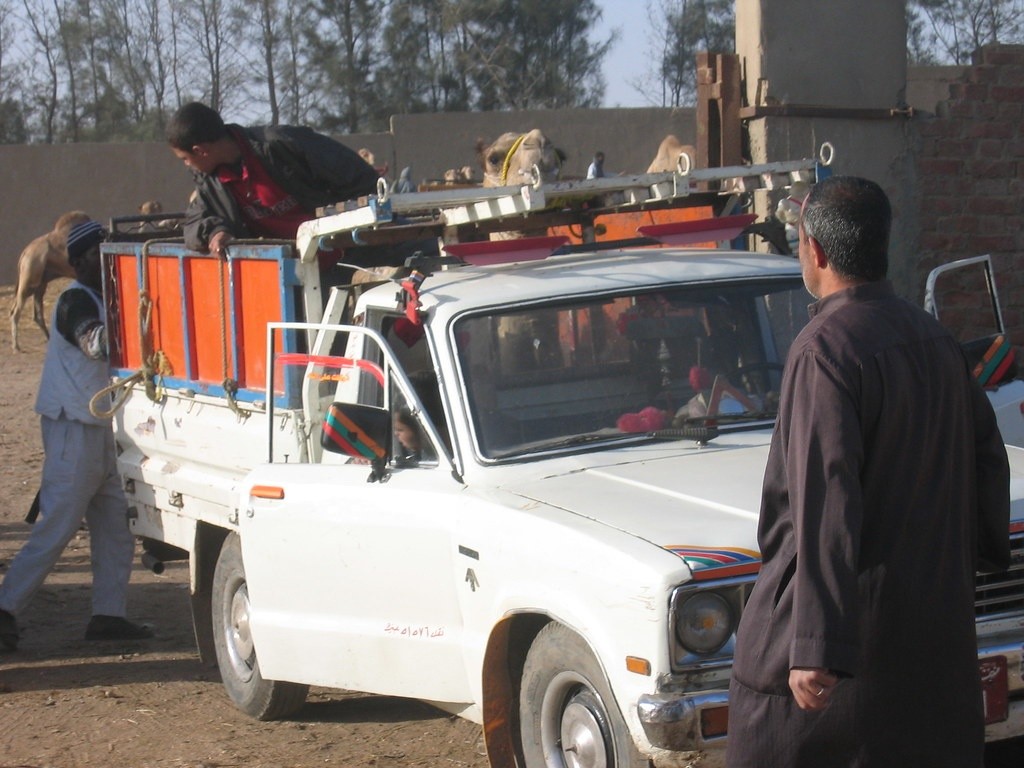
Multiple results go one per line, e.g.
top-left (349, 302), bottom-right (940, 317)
top-left (0, 610), bottom-right (21, 651)
top-left (85, 615), bottom-right (153, 641)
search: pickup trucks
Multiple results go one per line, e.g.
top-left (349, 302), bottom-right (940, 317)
top-left (94, 157), bottom-right (1024, 768)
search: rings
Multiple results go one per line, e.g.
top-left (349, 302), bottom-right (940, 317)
top-left (815, 688), bottom-right (824, 697)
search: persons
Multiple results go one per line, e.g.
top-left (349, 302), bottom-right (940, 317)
top-left (354, 368), bottom-right (458, 467)
top-left (162, 103), bottom-right (398, 350)
top-left (726, 176), bottom-right (1010, 768)
top-left (585, 151), bottom-right (606, 180)
top-left (0, 220), bottom-right (151, 652)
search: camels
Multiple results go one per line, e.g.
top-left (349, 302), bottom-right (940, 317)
top-left (9, 128), bottom-right (698, 384)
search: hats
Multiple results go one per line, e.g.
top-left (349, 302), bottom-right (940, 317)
top-left (66, 220), bottom-right (109, 259)
